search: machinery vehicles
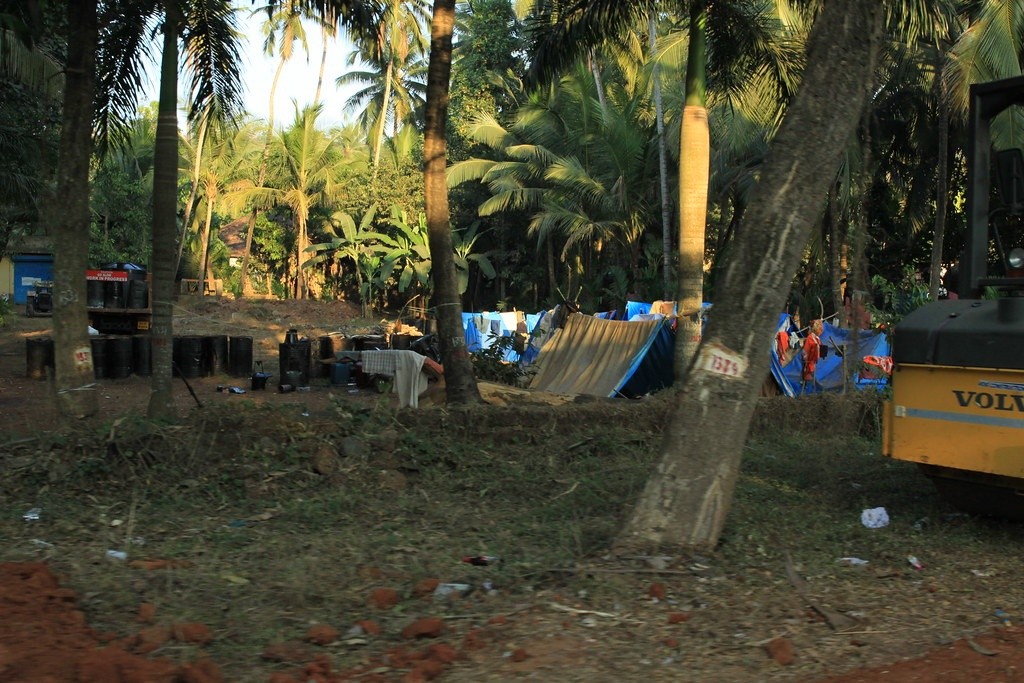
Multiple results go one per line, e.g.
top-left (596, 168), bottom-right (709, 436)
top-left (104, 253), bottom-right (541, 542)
top-left (878, 74), bottom-right (1024, 479)
top-left (26, 281), bottom-right (53, 317)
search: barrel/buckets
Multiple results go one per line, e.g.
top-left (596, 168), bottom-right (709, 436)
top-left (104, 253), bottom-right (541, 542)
top-left (87, 280), bottom-right (104, 307)
top-left (205, 335), bottom-right (228, 376)
top-left (127, 280), bottom-right (149, 309)
top-left (27, 334), bottom-right (55, 381)
top-left (182, 335), bottom-right (204, 377)
top-left (105, 282), bottom-right (124, 308)
top-left (229, 335), bottom-right (253, 377)
top-left (288, 342), bottom-right (311, 386)
top-left (88, 312), bottom-right (154, 380)
top-left (172, 335), bottom-right (183, 378)
top-left (279, 343), bottom-right (287, 386)
